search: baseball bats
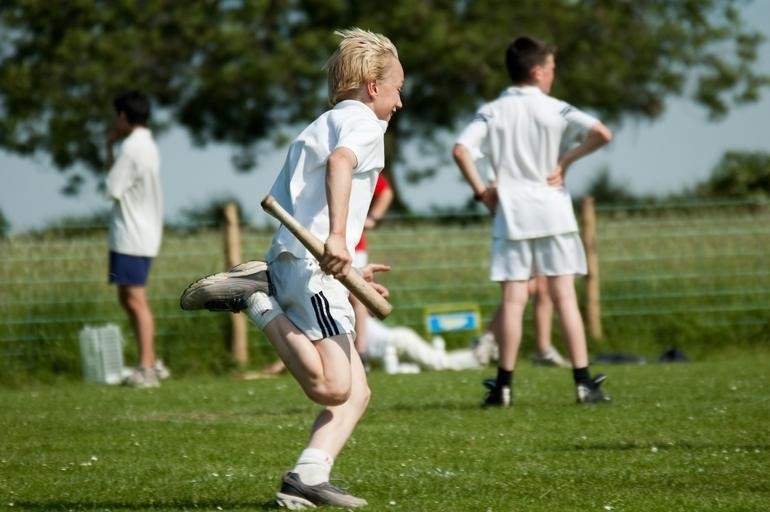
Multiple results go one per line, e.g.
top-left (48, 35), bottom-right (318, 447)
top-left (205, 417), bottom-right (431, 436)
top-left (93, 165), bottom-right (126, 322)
top-left (261, 195), bottom-right (393, 319)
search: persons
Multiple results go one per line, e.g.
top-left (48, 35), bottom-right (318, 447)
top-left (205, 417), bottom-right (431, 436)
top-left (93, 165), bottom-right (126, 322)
top-left (239, 172), bottom-right (395, 380)
top-left (177, 24), bottom-right (406, 510)
top-left (242, 282), bottom-right (500, 383)
top-left (453, 29), bottom-right (611, 406)
top-left (474, 275), bottom-right (570, 370)
top-left (99, 84), bottom-right (166, 389)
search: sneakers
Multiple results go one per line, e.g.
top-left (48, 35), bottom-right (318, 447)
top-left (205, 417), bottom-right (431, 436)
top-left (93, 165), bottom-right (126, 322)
top-left (276, 471), bottom-right (368, 510)
top-left (180, 261), bottom-right (276, 313)
top-left (576, 374), bottom-right (612, 402)
top-left (482, 379), bottom-right (512, 407)
